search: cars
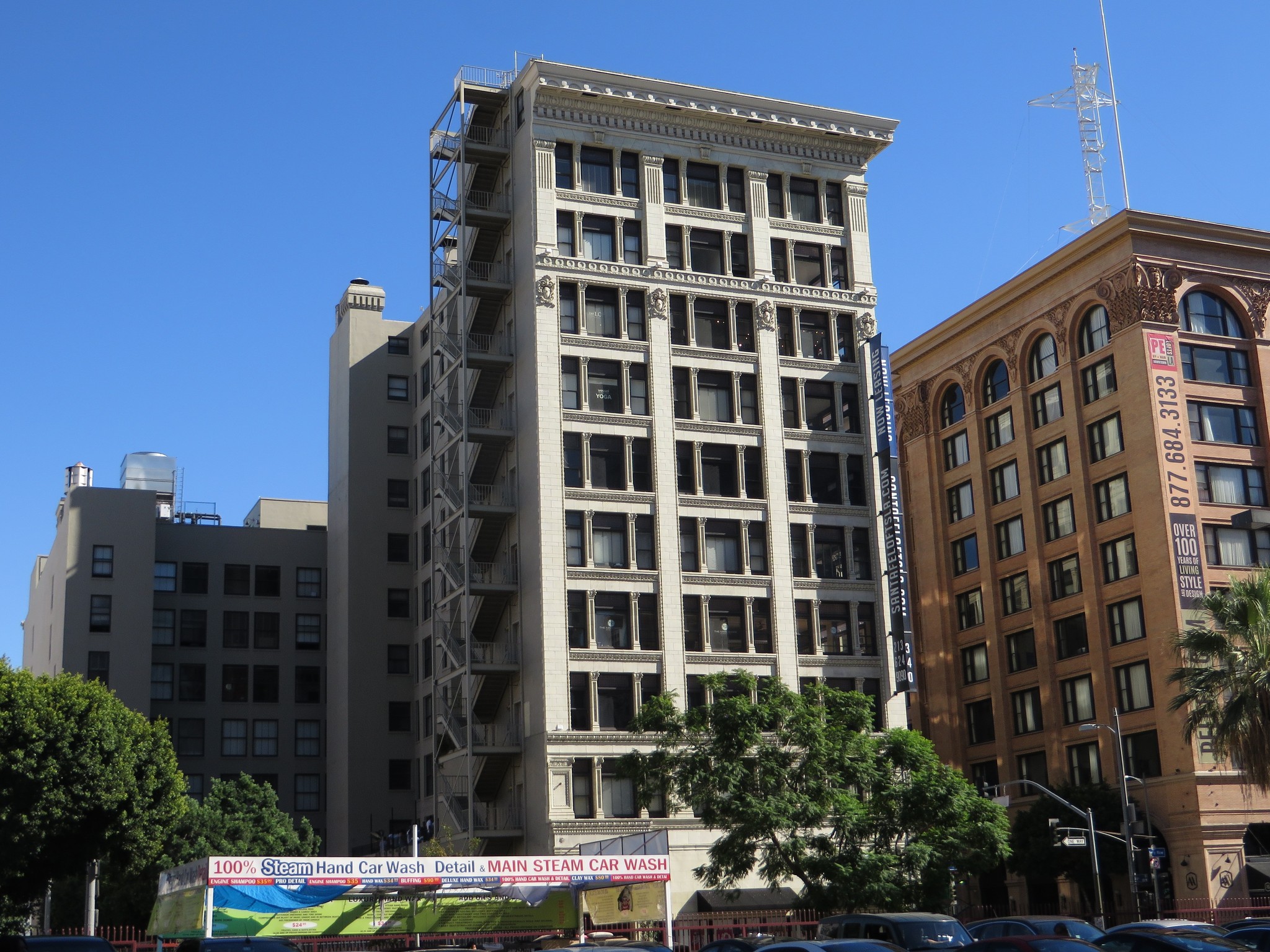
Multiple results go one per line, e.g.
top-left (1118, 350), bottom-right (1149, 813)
top-left (694, 934), bottom-right (912, 952)
top-left (1092, 916), bottom-right (1270, 952)
top-left (954, 934), bottom-right (1107, 952)
top-left (0, 933), bottom-right (121, 952)
top-left (172, 935), bottom-right (304, 952)
top-left (963, 915), bottom-right (1128, 952)
top-left (405, 931), bottom-right (677, 952)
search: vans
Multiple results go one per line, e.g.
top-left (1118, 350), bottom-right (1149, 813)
top-left (814, 911), bottom-right (978, 952)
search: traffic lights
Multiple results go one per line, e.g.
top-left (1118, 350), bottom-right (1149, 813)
top-left (950, 881), bottom-right (959, 905)
top-left (974, 775), bottom-right (989, 798)
top-left (1049, 818), bottom-right (1062, 847)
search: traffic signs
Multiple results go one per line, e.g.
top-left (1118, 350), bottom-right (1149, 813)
top-left (1061, 835), bottom-right (1088, 847)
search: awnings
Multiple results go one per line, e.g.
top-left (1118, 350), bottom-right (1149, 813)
top-left (1246, 861), bottom-right (1270, 892)
top-left (697, 887), bottom-right (802, 912)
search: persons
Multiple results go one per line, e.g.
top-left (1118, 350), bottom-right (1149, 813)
top-left (379, 815), bottom-right (434, 853)
top-left (466, 941), bottom-right (476, 950)
top-left (915, 927), bottom-right (964, 946)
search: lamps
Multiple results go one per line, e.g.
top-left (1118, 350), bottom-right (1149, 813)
top-left (1223, 852), bottom-right (1232, 864)
top-left (1010, 900), bottom-right (1016, 912)
top-left (1180, 854), bottom-right (1190, 867)
top-left (1209, 766), bottom-right (1217, 771)
top-left (1061, 896), bottom-right (1067, 909)
top-left (1175, 768), bottom-right (1181, 772)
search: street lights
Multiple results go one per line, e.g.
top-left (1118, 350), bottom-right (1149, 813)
top-left (1078, 706), bottom-right (1142, 921)
top-left (1125, 774), bottom-right (1162, 923)
